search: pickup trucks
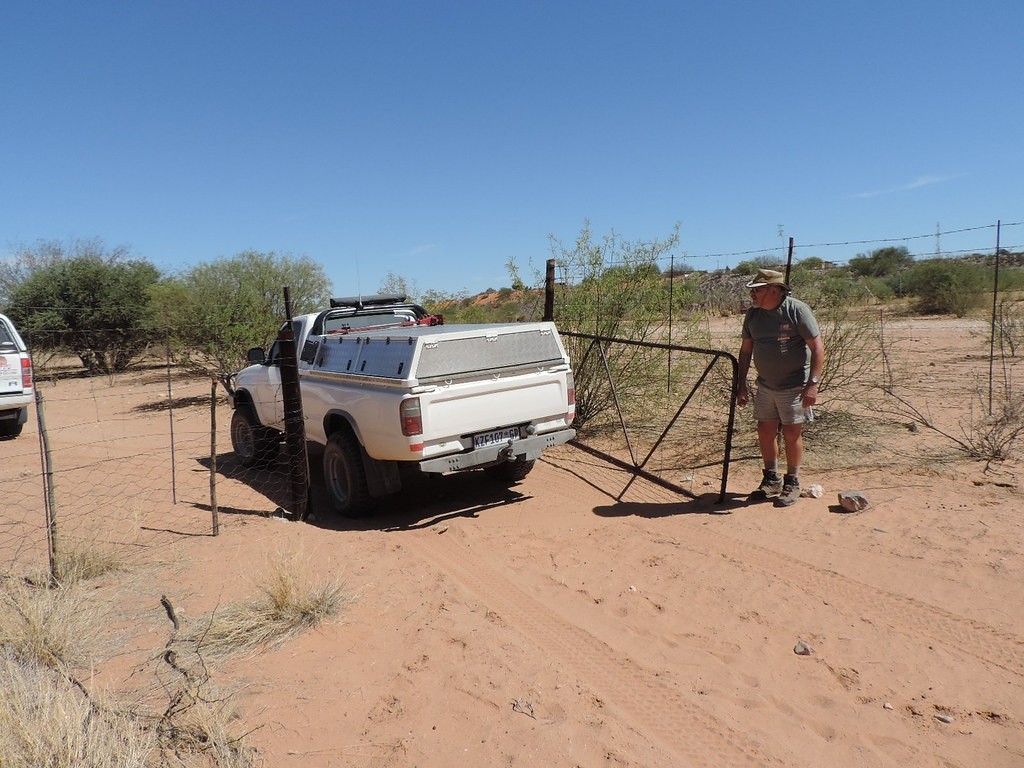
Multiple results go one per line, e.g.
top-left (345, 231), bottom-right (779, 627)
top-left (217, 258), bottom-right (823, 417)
top-left (230, 296), bottom-right (578, 518)
top-left (0, 314), bottom-right (34, 436)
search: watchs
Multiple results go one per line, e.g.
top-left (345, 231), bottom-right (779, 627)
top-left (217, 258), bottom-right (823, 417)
top-left (809, 376), bottom-right (818, 383)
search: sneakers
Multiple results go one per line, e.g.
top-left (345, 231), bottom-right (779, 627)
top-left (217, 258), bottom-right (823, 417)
top-left (750, 469), bottom-right (783, 500)
top-left (775, 473), bottom-right (802, 507)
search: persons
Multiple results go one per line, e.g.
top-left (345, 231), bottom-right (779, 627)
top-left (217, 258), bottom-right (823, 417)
top-left (737, 269), bottom-right (823, 505)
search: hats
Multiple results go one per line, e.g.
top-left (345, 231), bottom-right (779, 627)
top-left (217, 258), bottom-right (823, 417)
top-left (745, 269), bottom-right (792, 291)
